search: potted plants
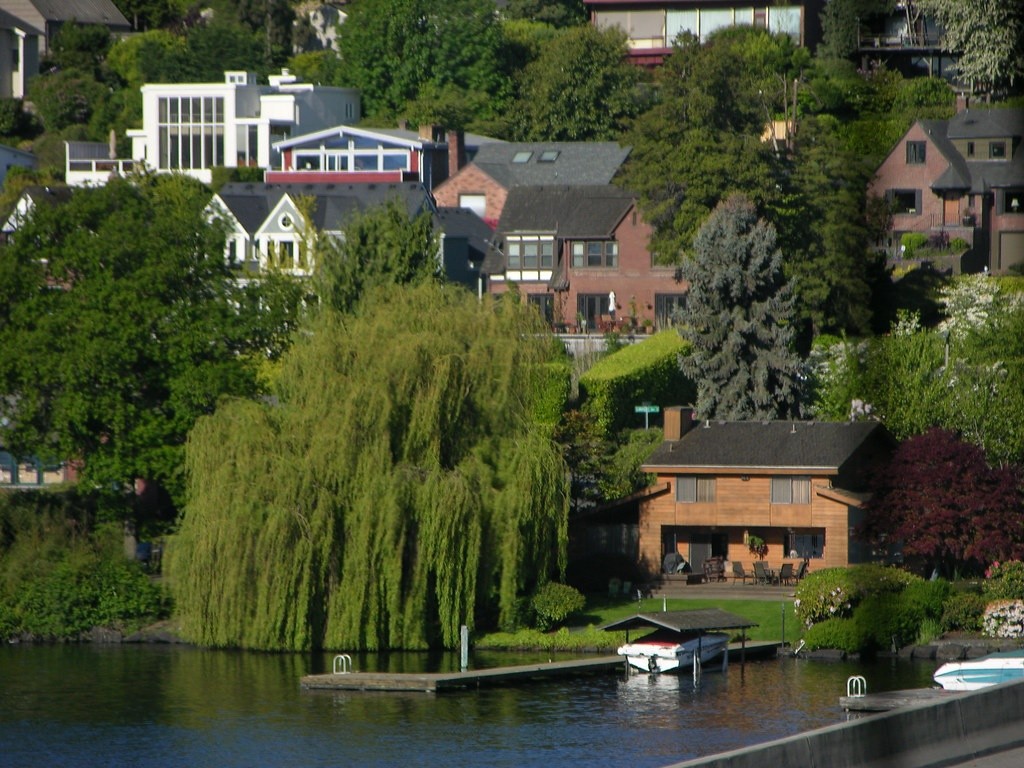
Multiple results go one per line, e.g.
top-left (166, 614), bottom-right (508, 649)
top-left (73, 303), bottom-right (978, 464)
top-left (642, 319), bottom-right (653, 334)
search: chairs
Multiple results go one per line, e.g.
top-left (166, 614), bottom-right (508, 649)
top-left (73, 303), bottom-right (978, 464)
top-left (702, 557), bottom-right (723, 582)
top-left (730, 561), bottom-right (809, 588)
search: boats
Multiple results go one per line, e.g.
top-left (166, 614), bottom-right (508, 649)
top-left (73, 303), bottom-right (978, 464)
top-left (617, 628), bottom-right (731, 672)
top-left (934, 649), bottom-right (1024, 691)
